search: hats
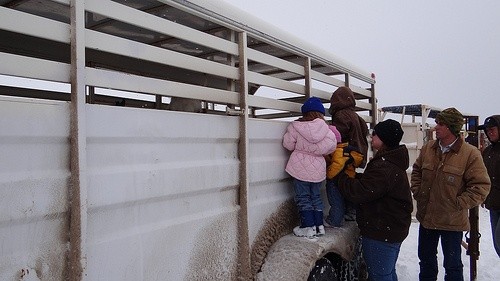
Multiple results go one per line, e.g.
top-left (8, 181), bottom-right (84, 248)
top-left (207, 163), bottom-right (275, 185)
top-left (328, 125), bottom-right (341, 143)
top-left (435, 108), bottom-right (464, 137)
top-left (374, 119), bottom-right (404, 145)
top-left (483, 115), bottom-right (500, 142)
top-left (301, 96), bottom-right (326, 115)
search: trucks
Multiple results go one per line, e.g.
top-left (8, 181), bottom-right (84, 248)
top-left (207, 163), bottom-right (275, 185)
top-left (0, 0), bottom-right (379, 281)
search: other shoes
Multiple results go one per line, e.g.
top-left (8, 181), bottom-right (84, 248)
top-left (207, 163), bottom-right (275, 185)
top-left (292, 225), bottom-right (325, 237)
top-left (323, 218), bottom-right (345, 228)
top-left (344, 213), bottom-right (356, 221)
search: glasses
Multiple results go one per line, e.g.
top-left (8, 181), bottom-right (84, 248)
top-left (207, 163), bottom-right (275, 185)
top-left (372, 131), bottom-right (377, 136)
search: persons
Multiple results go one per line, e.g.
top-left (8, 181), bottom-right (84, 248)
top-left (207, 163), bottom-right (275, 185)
top-left (411, 107), bottom-right (491, 281)
top-left (337, 119), bottom-right (414, 281)
top-left (282, 96), bottom-right (336, 237)
top-left (323, 87), bottom-right (371, 228)
top-left (474, 114), bottom-right (500, 257)
